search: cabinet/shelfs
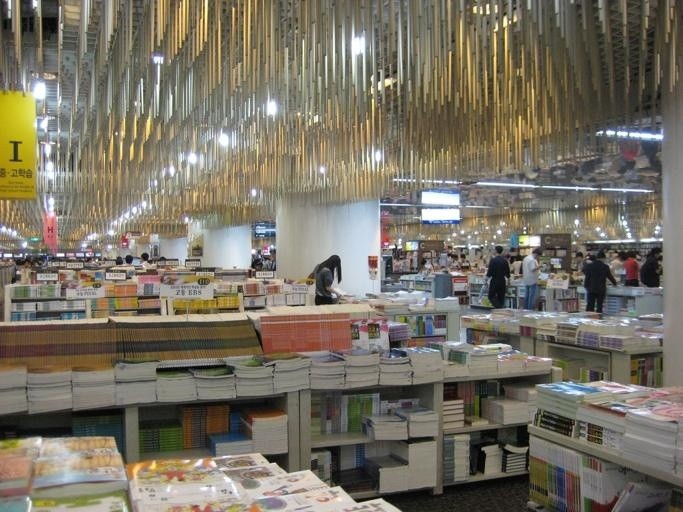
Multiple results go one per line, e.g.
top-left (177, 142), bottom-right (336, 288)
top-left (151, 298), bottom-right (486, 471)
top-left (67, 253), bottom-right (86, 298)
top-left (532, 338), bottom-right (662, 387)
top-left (524, 419), bottom-right (682, 512)
top-left (517, 233), bottom-right (573, 274)
top-left (399, 279), bottom-right (433, 296)
top-left (581, 242), bottom-right (662, 263)
top-left (124, 388), bottom-right (299, 476)
top-left (168, 290), bottom-right (312, 314)
top-left (407, 241), bottom-right (443, 272)
top-left (465, 327), bottom-right (535, 357)
top-left (3, 285), bottom-right (167, 322)
top-left (299, 366), bottom-right (564, 498)
top-left (467, 282), bottom-right (556, 312)
top-left (577, 286), bottom-right (662, 315)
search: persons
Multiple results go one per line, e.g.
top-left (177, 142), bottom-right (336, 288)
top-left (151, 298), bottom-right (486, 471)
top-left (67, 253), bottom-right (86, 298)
top-left (583, 249), bottom-right (618, 313)
top-left (639, 247), bottom-right (662, 288)
top-left (116, 252), bottom-right (149, 268)
top-left (522, 249), bottom-right (544, 310)
top-left (610, 251), bottom-right (639, 287)
top-left (252, 248), bottom-right (276, 276)
top-left (485, 246), bottom-right (510, 309)
top-left (512, 256), bottom-right (522, 274)
top-left (571, 252), bottom-right (586, 273)
top-left (312, 255), bottom-right (342, 306)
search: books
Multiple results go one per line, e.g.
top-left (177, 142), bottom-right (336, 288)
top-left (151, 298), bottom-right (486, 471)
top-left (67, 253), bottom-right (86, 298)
top-left (0, 269), bottom-right (317, 320)
top-left (363, 293), bottom-right (463, 315)
top-left (566, 313), bottom-right (668, 386)
top-left (443, 380), bottom-right (534, 487)
top-left (1, 322), bottom-right (562, 412)
top-left (0, 434), bottom-right (407, 508)
top-left (311, 388), bottom-right (437, 494)
top-left (544, 278), bottom-right (586, 311)
top-left (376, 272), bottom-right (435, 294)
top-left (74, 405), bottom-right (288, 454)
top-left (528, 380), bottom-right (681, 508)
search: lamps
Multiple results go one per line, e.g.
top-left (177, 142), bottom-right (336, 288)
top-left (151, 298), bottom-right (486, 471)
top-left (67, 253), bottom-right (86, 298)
top-left (421, 189), bottom-right (461, 205)
top-left (420, 208), bottom-right (463, 225)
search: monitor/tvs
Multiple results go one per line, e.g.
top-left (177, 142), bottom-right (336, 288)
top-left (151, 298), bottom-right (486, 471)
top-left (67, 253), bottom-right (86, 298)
top-left (420, 188), bottom-right (462, 225)
